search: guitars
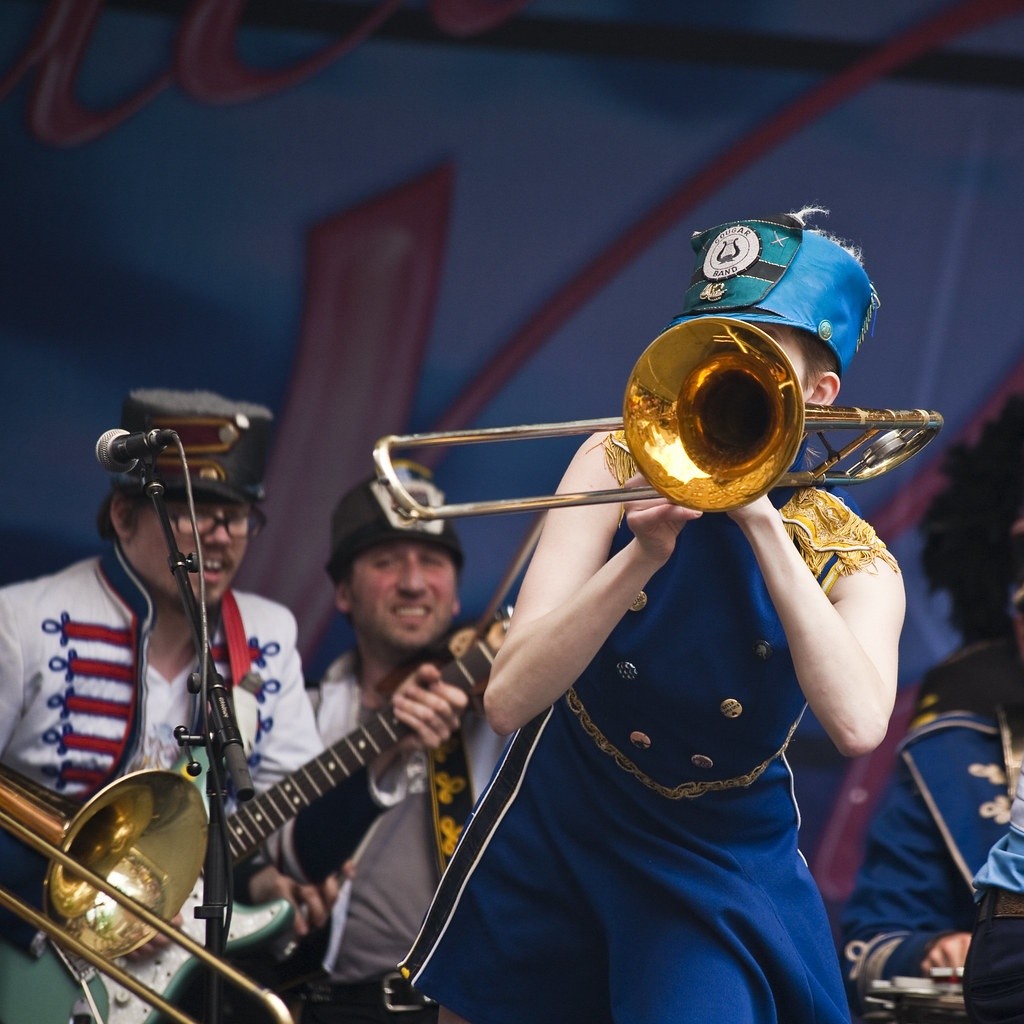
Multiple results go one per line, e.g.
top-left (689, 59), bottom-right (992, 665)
top-left (0, 611), bottom-right (508, 1022)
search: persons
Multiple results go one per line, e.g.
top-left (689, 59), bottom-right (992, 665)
top-left (0, 386), bottom-right (359, 1024)
top-left (962, 760), bottom-right (1024, 1024)
top-left (835, 388), bottom-right (1024, 1015)
top-left (395, 204), bottom-right (909, 1022)
top-left (225, 470), bottom-right (515, 1022)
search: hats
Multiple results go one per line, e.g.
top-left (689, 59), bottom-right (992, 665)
top-left (661, 216), bottom-right (875, 377)
top-left (117, 382), bottom-right (273, 502)
top-left (327, 479), bottom-right (464, 583)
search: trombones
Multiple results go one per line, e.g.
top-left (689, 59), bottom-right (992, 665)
top-left (366, 320), bottom-right (938, 540)
top-left (0, 765), bottom-right (297, 1023)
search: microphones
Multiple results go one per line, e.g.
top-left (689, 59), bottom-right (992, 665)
top-left (95, 428), bottom-right (177, 474)
